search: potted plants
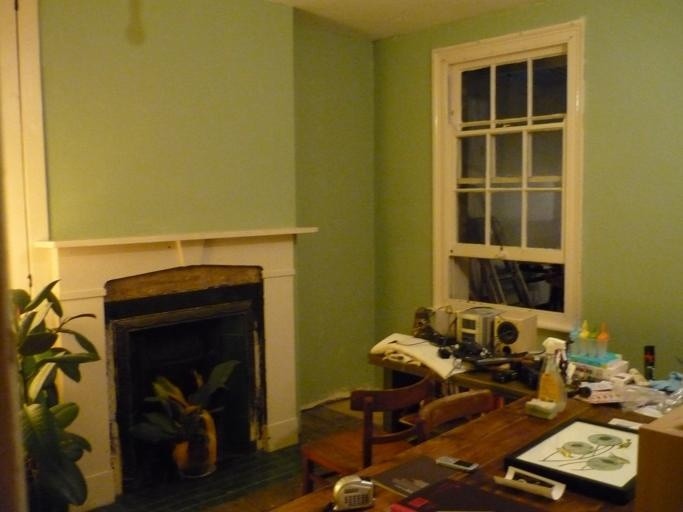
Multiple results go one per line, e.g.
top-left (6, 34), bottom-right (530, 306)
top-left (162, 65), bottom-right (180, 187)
top-left (124, 356), bottom-right (242, 481)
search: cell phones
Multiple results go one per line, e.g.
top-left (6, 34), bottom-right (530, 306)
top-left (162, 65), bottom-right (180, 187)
top-left (435, 454), bottom-right (480, 473)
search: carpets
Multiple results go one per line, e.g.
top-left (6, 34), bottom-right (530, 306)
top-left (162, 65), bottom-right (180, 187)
top-left (101, 404), bottom-right (372, 512)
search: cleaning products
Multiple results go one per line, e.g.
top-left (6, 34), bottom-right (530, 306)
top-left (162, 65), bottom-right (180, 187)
top-left (538, 337), bottom-right (569, 414)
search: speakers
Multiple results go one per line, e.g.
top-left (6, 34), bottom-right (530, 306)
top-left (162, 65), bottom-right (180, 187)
top-left (494, 310), bottom-right (538, 356)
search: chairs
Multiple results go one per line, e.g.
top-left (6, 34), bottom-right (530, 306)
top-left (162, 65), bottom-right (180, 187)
top-left (304, 374), bottom-right (495, 495)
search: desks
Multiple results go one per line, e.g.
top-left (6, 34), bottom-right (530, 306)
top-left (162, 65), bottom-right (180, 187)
top-left (369, 331), bottom-right (539, 396)
top-left (270, 392), bottom-right (662, 512)
